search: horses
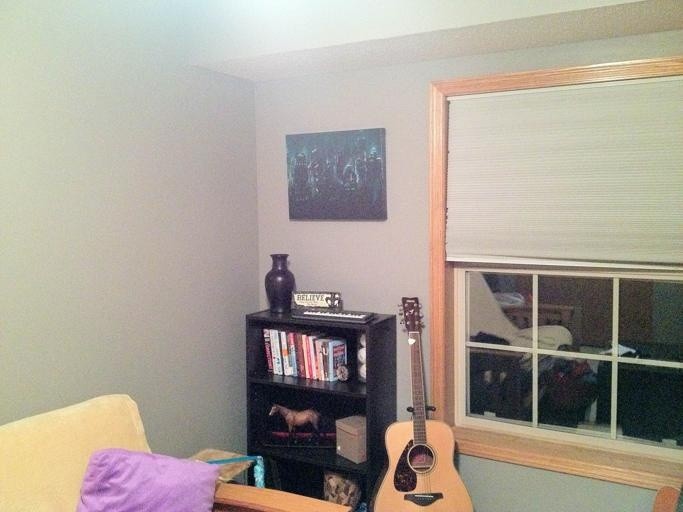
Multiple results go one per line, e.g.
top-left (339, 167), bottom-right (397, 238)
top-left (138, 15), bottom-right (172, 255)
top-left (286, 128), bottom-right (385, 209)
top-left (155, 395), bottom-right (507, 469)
top-left (267, 399), bottom-right (325, 446)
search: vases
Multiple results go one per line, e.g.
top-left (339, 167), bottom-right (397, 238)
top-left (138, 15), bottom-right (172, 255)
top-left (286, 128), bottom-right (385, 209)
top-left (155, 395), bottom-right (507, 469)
top-left (265, 253), bottom-right (295, 314)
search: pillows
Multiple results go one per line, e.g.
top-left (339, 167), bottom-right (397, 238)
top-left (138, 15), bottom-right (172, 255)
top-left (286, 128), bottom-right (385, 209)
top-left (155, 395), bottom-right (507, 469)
top-left (77, 449), bottom-right (220, 512)
top-left (0, 393), bottom-right (154, 512)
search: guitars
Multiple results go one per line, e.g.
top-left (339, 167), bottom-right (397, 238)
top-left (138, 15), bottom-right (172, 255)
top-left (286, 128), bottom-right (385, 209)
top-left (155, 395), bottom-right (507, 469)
top-left (371, 297), bottom-right (474, 511)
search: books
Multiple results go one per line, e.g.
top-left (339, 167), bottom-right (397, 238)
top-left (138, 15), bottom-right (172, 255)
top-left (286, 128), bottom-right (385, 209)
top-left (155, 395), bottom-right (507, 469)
top-left (262, 327), bottom-right (347, 383)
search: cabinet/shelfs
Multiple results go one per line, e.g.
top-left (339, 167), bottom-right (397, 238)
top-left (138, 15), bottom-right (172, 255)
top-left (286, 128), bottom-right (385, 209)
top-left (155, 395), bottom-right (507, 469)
top-left (246, 306), bottom-right (397, 511)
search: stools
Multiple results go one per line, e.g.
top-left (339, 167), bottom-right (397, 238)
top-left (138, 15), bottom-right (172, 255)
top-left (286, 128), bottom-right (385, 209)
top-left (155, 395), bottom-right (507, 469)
top-left (185, 449), bottom-right (257, 486)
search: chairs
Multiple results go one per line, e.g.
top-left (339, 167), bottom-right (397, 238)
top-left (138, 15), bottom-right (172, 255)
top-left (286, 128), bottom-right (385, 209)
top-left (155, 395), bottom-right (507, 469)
top-left (469, 272), bottom-right (575, 420)
top-left (213, 482), bottom-right (353, 511)
